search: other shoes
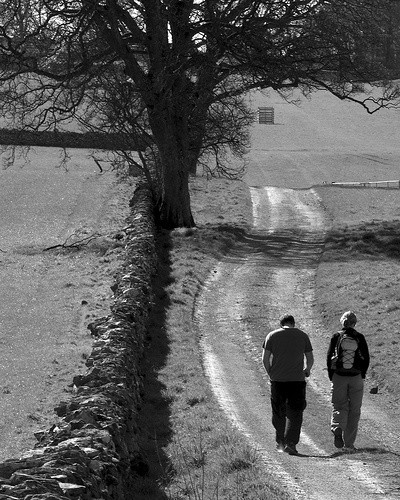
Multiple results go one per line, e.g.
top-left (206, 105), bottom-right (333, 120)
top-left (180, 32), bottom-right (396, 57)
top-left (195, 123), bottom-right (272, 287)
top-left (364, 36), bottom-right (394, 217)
top-left (342, 444), bottom-right (358, 454)
top-left (275, 442), bottom-right (285, 450)
top-left (284, 444), bottom-right (299, 455)
top-left (333, 427), bottom-right (344, 448)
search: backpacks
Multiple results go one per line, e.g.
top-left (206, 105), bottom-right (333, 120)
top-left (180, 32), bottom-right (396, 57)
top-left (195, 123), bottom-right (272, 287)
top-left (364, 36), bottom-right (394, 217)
top-left (329, 330), bottom-right (366, 376)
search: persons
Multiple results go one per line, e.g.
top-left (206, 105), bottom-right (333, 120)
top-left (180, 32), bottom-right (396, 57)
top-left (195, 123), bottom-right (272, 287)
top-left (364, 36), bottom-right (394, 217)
top-left (262, 314), bottom-right (314, 455)
top-left (327, 311), bottom-right (370, 450)
